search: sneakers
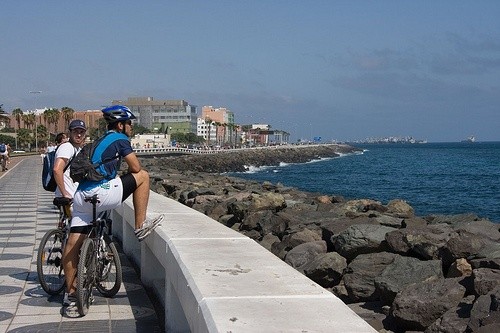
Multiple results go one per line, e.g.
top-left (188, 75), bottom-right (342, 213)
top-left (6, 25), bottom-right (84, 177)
top-left (63, 291), bottom-right (77, 306)
top-left (133, 214), bottom-right (166, 242)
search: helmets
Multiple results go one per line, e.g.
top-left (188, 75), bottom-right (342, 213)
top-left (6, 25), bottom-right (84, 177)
top-left (101, 105), bottom-right (137, 122)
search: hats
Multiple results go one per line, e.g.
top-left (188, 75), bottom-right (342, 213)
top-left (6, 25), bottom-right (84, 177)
top-left (68, 120), bottom-right (87, 130)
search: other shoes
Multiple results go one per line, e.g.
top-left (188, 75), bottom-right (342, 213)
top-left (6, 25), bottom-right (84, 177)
top-left (5, 168), bottom-right (7, 170)
top-left (2, 168), bottom-right (5, 171)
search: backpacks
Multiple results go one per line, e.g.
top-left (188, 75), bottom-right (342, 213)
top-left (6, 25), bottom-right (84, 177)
top-left (69, 132), bottom-right (120, 182)
top-left (42, 150), bottom-right (58, 192)
top-left (0, 144), bottom-right (5, 152)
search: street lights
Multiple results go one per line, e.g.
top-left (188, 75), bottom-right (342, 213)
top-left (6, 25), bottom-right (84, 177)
top-left (30, 90), bottom-right (41, 152)
top-left (14, 106), bottom-right (19, 150)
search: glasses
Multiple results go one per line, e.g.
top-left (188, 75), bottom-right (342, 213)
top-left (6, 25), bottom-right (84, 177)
top-left (125, 121), bottom-right (132, 125)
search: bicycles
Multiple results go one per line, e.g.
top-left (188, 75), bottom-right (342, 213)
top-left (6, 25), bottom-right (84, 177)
top-left (37, 194), bottom-right (123, 316)
top-left (0, 154), bottom-right (8, 172)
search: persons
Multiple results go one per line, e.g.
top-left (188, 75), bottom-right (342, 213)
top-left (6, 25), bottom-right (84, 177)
top-left (61, 106), bottom-right (164, 305)
top-left (46, 133), bottom-right (67, 152)
top-left (0, 141), bottom-right (11, 170)
top-left (53, 120), bottom-right (87, 240)
